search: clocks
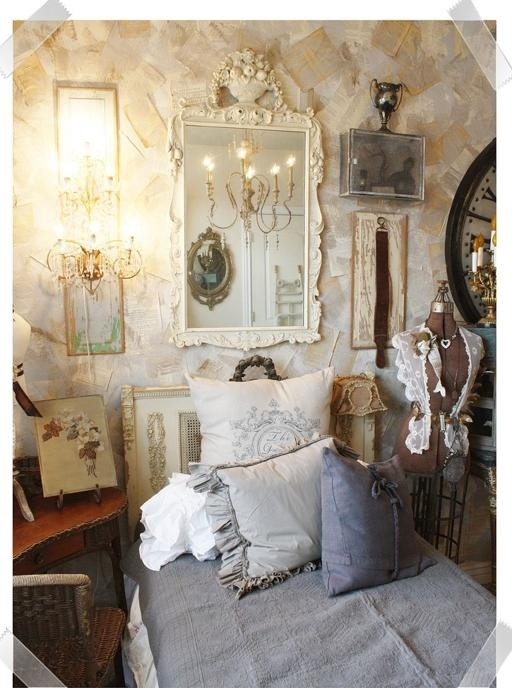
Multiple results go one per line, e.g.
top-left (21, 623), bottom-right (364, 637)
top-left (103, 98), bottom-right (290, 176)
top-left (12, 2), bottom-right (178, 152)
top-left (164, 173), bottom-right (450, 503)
top-left (444, 135), bottom-right (496, 330)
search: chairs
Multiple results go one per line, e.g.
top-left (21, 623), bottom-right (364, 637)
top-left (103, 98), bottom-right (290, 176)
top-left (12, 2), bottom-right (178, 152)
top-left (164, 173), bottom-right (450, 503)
top-left (14, 572), bottom-right (127, 687)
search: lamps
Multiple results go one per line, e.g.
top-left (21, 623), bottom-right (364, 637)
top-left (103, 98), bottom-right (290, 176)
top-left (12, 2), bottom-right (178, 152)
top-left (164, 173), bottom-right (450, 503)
top-left (45, 119), bottom-right (143, 293)
top-left (203, 125), bottom-right (296, 234)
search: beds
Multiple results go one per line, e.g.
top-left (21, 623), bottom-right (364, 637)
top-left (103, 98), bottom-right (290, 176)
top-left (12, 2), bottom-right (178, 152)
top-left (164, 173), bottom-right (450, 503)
top-left (119, 353), bottom-right (496, 688)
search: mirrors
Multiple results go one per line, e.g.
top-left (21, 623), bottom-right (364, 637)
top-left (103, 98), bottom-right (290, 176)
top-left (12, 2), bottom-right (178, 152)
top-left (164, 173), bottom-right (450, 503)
top-left (188, 239), bottom-right (231, 297)
top-left (163, 100), bottom-right (324, 351)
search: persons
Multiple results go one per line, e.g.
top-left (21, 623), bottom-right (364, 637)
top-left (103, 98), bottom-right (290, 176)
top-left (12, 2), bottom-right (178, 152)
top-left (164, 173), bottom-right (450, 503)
top-left (389, 294), bottom-right (485, 481)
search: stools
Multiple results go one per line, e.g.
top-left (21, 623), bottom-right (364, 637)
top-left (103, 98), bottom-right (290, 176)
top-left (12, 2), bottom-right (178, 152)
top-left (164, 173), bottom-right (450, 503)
top-left (412, 471), bottom-right (468, 569)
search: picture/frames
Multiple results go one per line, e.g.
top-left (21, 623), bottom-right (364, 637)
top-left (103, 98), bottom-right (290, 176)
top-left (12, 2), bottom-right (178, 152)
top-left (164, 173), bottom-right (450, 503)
top-left (64, 272), bottom-right (126, 358)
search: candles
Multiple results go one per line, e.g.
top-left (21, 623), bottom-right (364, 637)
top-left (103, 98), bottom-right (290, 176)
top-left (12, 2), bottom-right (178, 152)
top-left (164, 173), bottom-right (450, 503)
top-left (472, 211), bottom-right (497, 278)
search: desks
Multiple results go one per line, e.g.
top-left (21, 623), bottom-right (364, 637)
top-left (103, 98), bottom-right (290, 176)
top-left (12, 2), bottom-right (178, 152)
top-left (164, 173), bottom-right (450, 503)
top-left (13, 472), bottom-right (128, 611)
